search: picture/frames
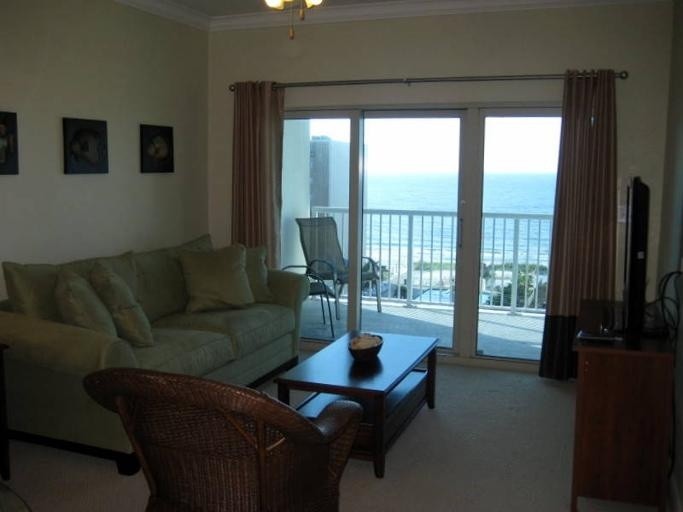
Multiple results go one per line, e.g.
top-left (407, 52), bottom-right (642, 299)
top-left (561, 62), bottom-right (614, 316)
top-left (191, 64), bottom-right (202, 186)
top-left (139, 123), bottom-right (175, 174)
top-left (62, 116), bottom-right (109, 175)
top-left (0, 109), bottom-right (20, 174)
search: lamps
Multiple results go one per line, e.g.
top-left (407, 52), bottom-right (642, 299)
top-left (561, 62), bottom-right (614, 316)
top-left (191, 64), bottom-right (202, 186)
top-left (264, 0), bottom-right (323, 40)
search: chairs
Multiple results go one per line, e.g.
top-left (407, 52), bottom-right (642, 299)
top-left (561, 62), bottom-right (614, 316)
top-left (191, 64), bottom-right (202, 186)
top-left (295, 216), bottom-right (382, 320)
top-left (84, 365), bottom-right (363, 512)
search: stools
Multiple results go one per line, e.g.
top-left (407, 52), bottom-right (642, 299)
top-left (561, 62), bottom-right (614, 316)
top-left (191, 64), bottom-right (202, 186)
top-left (280, 264), bottom-right (335, 337)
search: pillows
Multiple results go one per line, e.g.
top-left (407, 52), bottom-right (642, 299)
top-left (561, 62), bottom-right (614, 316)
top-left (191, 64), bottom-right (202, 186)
top-left (88, 259), bottom-right (154, 347)
top-left (53, 270), bottom-right (117, 338)
top-left (244, 244), bottom-right (275, 303)
top-left (179, 243), bottom-right (255, 313)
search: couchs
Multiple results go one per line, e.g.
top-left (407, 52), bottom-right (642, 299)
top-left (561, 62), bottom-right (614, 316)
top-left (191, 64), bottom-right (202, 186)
top-left (0, 235), bottom-right (311, 475)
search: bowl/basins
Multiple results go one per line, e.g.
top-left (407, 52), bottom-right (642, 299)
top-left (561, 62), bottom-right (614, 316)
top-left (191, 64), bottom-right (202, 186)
top-left (348, 334), bottom-right (383, 360)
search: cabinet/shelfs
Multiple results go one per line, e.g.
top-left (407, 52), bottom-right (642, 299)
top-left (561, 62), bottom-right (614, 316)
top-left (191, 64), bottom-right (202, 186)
top-left (569, 312), bottom-right (674, 511)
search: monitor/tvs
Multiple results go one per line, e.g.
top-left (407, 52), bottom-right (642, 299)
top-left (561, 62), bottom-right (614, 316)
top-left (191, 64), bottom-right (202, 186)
top-left (611, 174), bottom-right (654, 351)
